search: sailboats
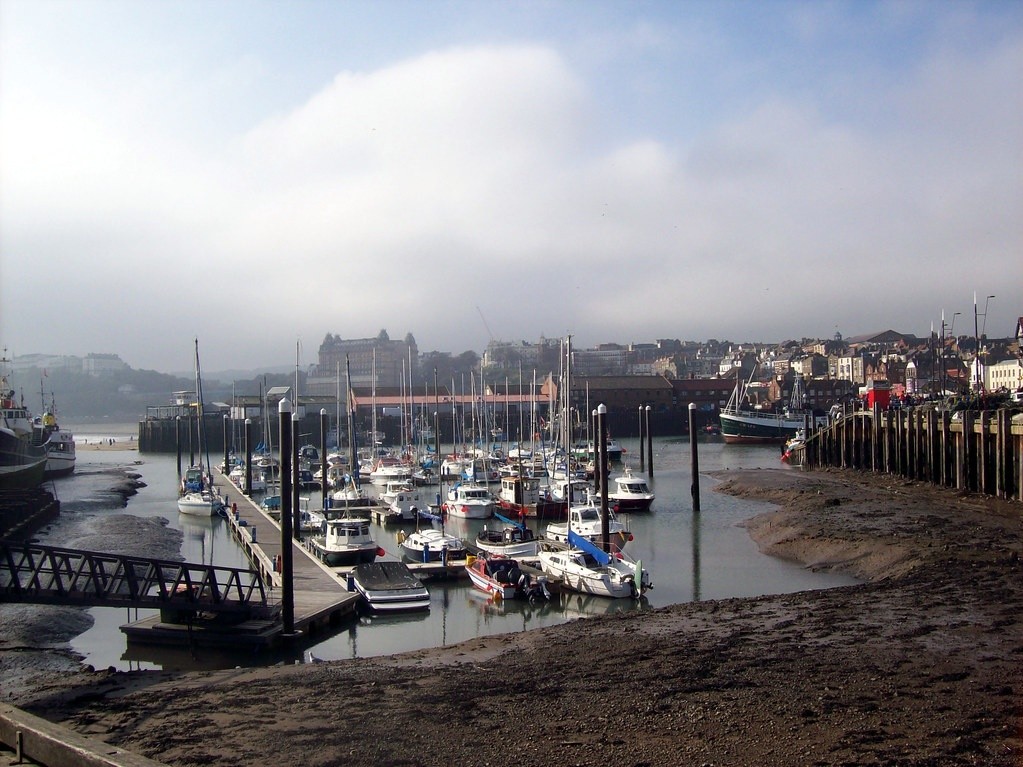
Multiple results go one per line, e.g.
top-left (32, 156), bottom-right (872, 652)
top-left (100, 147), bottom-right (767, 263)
top-left (473, 426), bottom-right (546, 556)
top-left (176, 338), bottom-right (654, 519)
top-left (399, 368), bottom-right (468, 562)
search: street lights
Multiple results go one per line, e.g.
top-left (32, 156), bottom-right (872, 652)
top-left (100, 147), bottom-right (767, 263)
top-left (978, 295), bottom-right (997, 381)
top-left (949, 311), bottom-right (961, 342)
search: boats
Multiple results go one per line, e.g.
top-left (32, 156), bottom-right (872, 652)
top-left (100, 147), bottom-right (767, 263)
top-left (467, 587), bottom-right (552, 615)
top-left (354, 560), bottom-right (432, 613)
top-left (466, 554), bottom-right (563, 601)
top-left (303, 517), bottom-right (385, 567)
top-left (537, 533), bottom-right (655, 601)
top-left (0, 345), bottom-right (76, 477)
top-left (546, 489), bottom-right (633, 557)
top-left (557, 587), bottom-right (650, 619)
top-left (717, 359), bottom-right (829, 445)
